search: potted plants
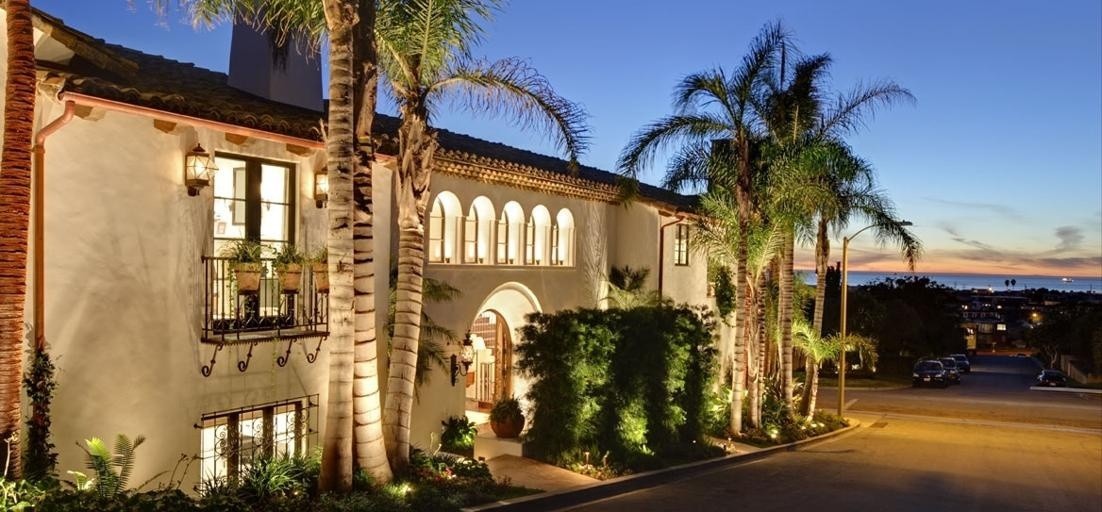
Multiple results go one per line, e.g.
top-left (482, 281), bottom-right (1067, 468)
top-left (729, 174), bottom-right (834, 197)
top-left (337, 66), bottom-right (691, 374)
top-left (270, 244), bottom-right (310, 295)
top-left (308, 245), bottom-right (334, 291)
top-left (486, 396), bottom-right (526, 440)
top-left (439, 413), bottom-right (477, 456)
top-left (216, 237), bottom-right (276, 292)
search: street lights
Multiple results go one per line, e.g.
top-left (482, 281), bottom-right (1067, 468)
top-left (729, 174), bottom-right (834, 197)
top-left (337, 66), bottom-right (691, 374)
top-left (836, 218), bottom-right (913, 420)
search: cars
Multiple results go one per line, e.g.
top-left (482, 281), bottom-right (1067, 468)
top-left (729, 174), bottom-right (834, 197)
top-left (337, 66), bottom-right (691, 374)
top-left (1035, 369), bottom-right (1071, 387)
top-left (1007, 352), bottom-right (1032, 359)
top-left (949, 352), bottom-right (972, 372)
top-left (911, 358), bottom-right (948, 389)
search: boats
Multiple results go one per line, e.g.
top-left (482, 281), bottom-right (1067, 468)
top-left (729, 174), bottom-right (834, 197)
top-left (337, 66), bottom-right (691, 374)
top-left (1062, 276), bottom-right (1074, 283)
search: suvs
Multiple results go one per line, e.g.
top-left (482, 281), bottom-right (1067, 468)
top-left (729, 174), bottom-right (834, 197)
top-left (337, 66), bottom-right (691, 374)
top-left (935, 357), bottom-right (961, 384)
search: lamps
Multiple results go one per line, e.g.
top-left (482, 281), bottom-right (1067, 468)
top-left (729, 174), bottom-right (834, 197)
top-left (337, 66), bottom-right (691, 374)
top-left (184, 139), bottom-right (214, 198)
top-left (314, 159), bottom-right (330, 213)
